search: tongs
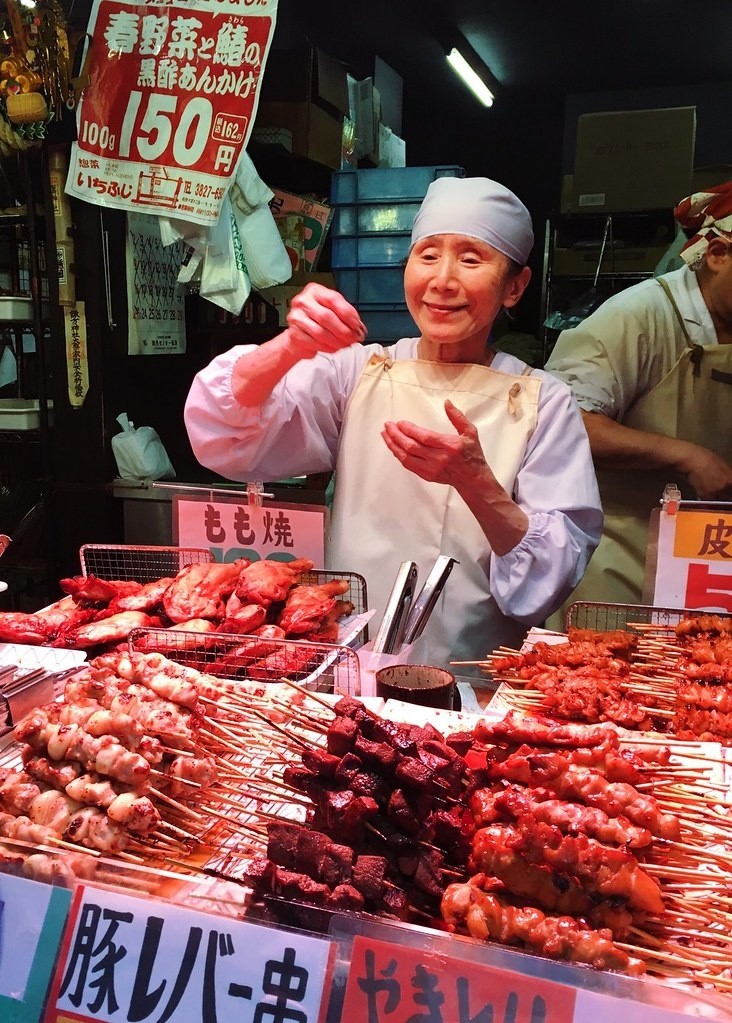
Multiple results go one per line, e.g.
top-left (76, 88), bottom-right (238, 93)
top-left (1, 665), bottom-right (89, 728)
top-left (402, 553), bottom-right (461, 645)
top-left (372, 560), bottom-right (419, 656)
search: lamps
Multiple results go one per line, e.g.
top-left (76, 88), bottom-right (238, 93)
top-left (445, 45), bottom-right (496, 108)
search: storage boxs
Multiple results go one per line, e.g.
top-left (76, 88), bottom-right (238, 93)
top-left (249, 37), bottom-right (349, 171)
top-left (0, 398), bottom-right (54, 429)
top-left (0, 296), bottom-right (52, 321)
top-left (331, 166), bottom-right (465, 349)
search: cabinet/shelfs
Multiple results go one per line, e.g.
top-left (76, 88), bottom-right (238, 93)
top-left (0, 147), bottom-right (55, 447)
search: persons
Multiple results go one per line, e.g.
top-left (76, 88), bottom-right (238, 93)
top-left (544, 182), bottom-right (732, 635)
top-left (183, 177), bottom-right (604, 677)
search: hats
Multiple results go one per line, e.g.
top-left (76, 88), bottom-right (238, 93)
top-left (409, 176), bottom-right (534, 266)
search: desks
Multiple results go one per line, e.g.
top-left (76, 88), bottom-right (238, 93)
top-left (112, 479), bottom-right (326, 586)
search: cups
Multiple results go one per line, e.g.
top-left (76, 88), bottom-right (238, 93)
top-left (375, 664), bottom-right (454, 712)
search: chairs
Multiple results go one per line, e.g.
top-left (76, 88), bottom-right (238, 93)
top-left (0, 465), bottom-right (54, 612)
